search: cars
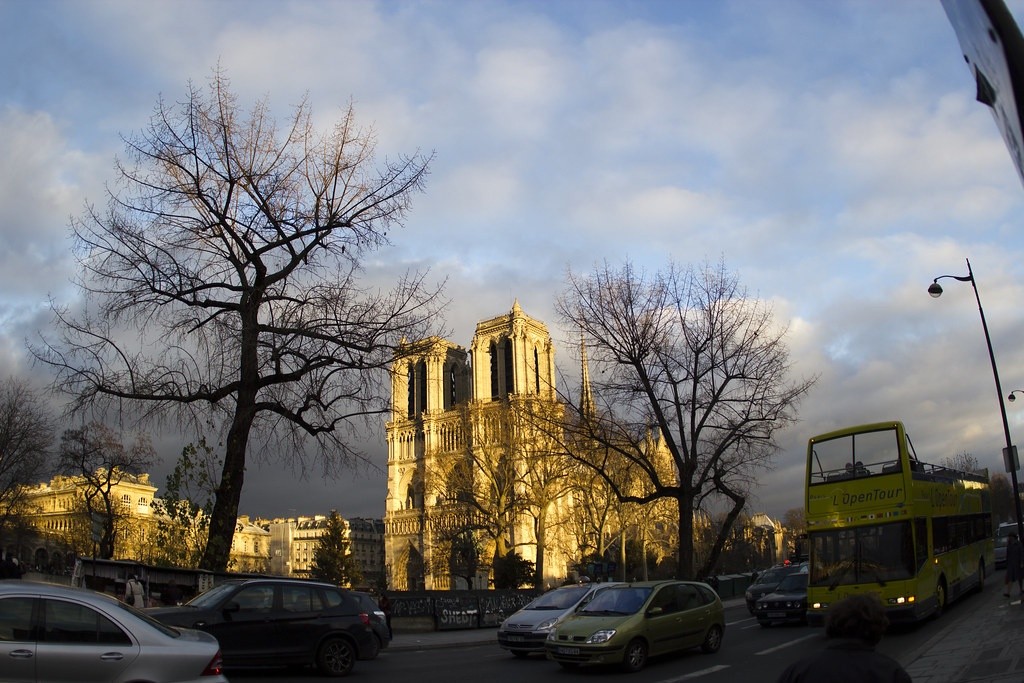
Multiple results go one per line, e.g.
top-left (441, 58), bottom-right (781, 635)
top-left (496, 583), bottom-right (621, 660)
top-left (344, 589), bottom-right (389, 660)
top-left (0, 578), bottom-right (228, 683)
top-left (545, 578), bottom-right (726, 673)
top-left (756, 573), bottom-right (825, 627)
top-left (993, 521), bottom-right (1021, 564)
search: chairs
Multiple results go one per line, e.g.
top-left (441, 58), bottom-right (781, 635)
top-left (293, 595), bottom-right (308, 611)
top-left (250, 591), bottom-right (270, 614)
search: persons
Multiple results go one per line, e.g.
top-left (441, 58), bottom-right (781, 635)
top-left (542, 573), bottom-right (721, 595)
top-left (121, 573), bottom-right (147, 610)
top-left (242, 591), bottom-right (269, 610)
top-left (750, 569), bottom-right (759, 584)
top-left (0, 550), bottom-right (74, 587)
top-left (998, 531), bottom-right (1024, 599)
top-left (839, 462), bottom-right (856, 477)
top-left (853, 461), bottom-right (868, 475)
top-left (158, 581), bottom-right (177, 606)
top-left (769, 587), bottom-right (914, 683)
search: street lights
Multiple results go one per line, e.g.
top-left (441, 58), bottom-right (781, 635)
top-left (926, 258), bottom-right (1024, 547)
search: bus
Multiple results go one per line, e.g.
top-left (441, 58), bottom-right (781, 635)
top-left (798, 421), bottom-right (997, 632)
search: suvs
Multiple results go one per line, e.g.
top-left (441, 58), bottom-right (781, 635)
top-left (139, 576), bottom-right (371, 678)
top-left (745, 562), bottom-right (810, 616)
